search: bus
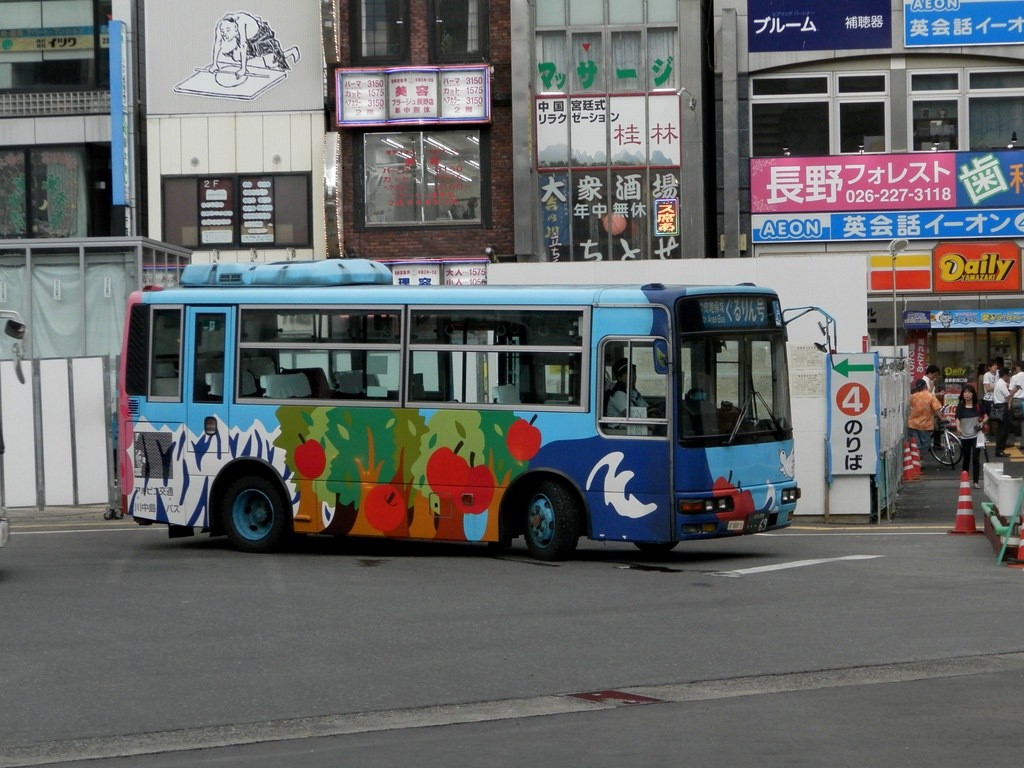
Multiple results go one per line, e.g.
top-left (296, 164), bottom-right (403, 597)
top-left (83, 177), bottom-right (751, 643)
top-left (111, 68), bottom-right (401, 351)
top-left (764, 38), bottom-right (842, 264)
top-left (116, 255), bottom-right (839, 562)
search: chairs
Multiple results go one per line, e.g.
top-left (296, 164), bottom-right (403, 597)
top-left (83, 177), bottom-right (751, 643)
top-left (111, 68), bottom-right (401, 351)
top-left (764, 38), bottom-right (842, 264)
top-left (493, 384), bottom-right (521, 405)
top-left (259, 374), bottom-right (312, 398)
top-left (282, 367), bottom-right (329, 393)
top-left (196, 360), bottom-right (223, 382)
top-left (412, 372), bottom-right (426, 401)
top-left (204, 373), bottom-right (257, 396)
top-left (339, 373), bottom-right (379, 394)
top-left (240, 356), bottom-right (275, 377)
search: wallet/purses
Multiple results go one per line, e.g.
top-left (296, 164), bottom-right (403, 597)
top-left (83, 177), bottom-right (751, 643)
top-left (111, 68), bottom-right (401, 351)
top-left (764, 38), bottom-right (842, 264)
top-left (974, 425), bottom-right (982, 432)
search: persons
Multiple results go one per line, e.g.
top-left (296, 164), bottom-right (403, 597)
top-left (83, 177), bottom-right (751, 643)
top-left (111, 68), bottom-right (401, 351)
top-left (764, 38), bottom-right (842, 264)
top-left (922, 365), bottom-right (946, 450)
top-left (907, 378), bottom-right (946, 468)
top-left (209, 11), bottom-right (301, 79)
top-left (983, 360), bottom-right (1001, 435)
top-left (954, 384), bottom-right (989, 489)
top-left (607, 356), bottom-right (660, 431)
top-left (993, 367), bottom-right (1021, 458)
top-left (1008, 360), bottom-right (1024, 449)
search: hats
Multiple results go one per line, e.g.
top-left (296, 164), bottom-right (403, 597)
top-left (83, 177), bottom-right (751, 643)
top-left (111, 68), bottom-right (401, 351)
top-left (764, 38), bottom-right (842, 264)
top-left (916, 379), bottom-right (926, 389)
top-left (612, 358), bottom-right (637, 379)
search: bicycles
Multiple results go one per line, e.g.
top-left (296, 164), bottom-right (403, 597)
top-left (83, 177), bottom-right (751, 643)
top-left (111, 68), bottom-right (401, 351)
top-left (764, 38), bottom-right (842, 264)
top-left (928, 404), bottom-right (963, 471)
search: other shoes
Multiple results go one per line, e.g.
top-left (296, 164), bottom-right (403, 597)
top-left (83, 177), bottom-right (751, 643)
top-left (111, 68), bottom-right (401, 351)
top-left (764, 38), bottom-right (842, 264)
top-left (995, 451), bottom-right (1011, 458)
top-left (974, 483), bottom-right (981, 489)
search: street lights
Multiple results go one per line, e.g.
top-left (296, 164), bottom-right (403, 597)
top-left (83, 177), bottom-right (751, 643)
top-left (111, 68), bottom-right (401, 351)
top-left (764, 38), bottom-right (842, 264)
top-left (888, 238), bottom-right (910, 347)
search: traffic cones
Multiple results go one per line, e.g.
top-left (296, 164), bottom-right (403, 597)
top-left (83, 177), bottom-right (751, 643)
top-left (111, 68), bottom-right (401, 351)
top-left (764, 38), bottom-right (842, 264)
top-left (902, 441), bottom-right (921, 484)
top-left (1007, 513), bottom-right (1024, 568)
top-left (947, 470), bottom-right (984, 534)
top-left (909, 437), bottom-right (926, 476)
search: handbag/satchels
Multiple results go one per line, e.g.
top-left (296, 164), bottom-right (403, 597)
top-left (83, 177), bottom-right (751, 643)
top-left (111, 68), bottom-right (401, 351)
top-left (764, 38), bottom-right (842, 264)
top-left (989, 401), bottom-right (1006, 422)
top-left (976, 424), bottom-right (986, 450)
top-left (976, 402), bottom-right (991, 434)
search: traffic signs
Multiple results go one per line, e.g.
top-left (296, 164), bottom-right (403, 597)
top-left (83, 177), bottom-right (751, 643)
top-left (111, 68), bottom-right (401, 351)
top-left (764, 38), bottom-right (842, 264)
top-left (825, 352), bottom-right (881, 484)
